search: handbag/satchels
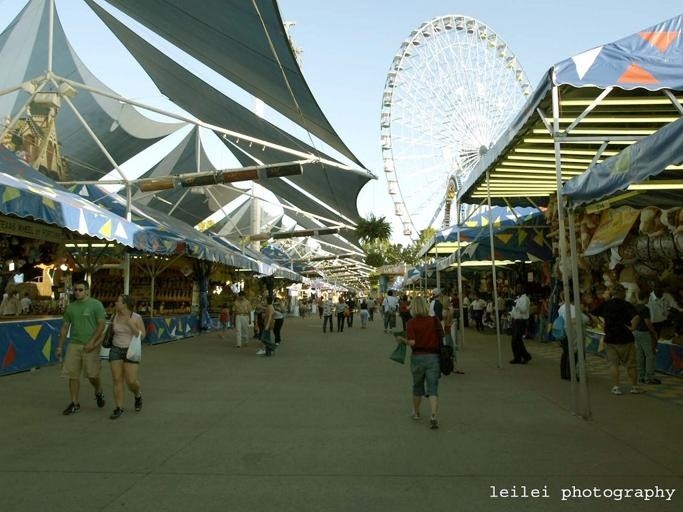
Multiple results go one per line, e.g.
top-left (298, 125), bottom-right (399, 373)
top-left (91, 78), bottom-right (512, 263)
top-left (126, 331), bottom-right (142, 362)
top-left (344, 308), bottom-right (349, 317)
top-left (437, 346), bottom-right (454, 376)
top-left (102, 312), bottom-right (115, 348)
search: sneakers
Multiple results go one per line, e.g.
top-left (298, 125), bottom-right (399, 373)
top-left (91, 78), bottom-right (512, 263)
top-left (645, 379), bottom-right (661, 384)
top-left (411, 411), bottom-right (421, 420)
top-left (630, 386), bottom-right (643, 394)
top-left (638, 377), bottom-right (645, 383)
top-left (611, 386), bottom-right (623, 395)
top-left (429, 414), bottom-right (440, 429)
top-left (234, 336), bottom-right (281, 357)
top-left (109, 406), bottom-right (124, 419)
top-left (63, 401), bottom-right (80, 415)
top-left (94, 391), bottom-right (105, 408)
top-left (134, 395), bottom-right (143, 411)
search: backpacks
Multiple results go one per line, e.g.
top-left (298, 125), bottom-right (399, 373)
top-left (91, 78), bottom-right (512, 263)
top-left (388, 306), bottom-right (396, 316)
top-left (552, 315), bottom-right (566, 340)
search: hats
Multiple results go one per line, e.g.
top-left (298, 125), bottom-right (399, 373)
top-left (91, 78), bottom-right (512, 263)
top-left (612, 284), bottom-right (628, 295)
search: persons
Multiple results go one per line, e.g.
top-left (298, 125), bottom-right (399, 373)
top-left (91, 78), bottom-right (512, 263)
top-left (0, 288), bottom-right (33, 317)
top-left (216, 289), bottom-right (284, 358)
top-left (396, 296), bottom-right (445, 429)
top-left (55, 280), bottom-right (106, 416)
top-left (108, 294), bottom-right (146, 419)
top-left (450, 279), bottom-right (683, 397)
top-left (299, 289), bottom-right (413, 333)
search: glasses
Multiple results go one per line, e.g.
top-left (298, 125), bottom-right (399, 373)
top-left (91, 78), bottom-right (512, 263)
top-left (75, 288), bottom-right (83, 291)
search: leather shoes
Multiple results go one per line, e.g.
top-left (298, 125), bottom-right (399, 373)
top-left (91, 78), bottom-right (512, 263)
top-left (522, 356), bottom-right (531, 364)
top-left (509, 360), bottom-right (521, 364)
